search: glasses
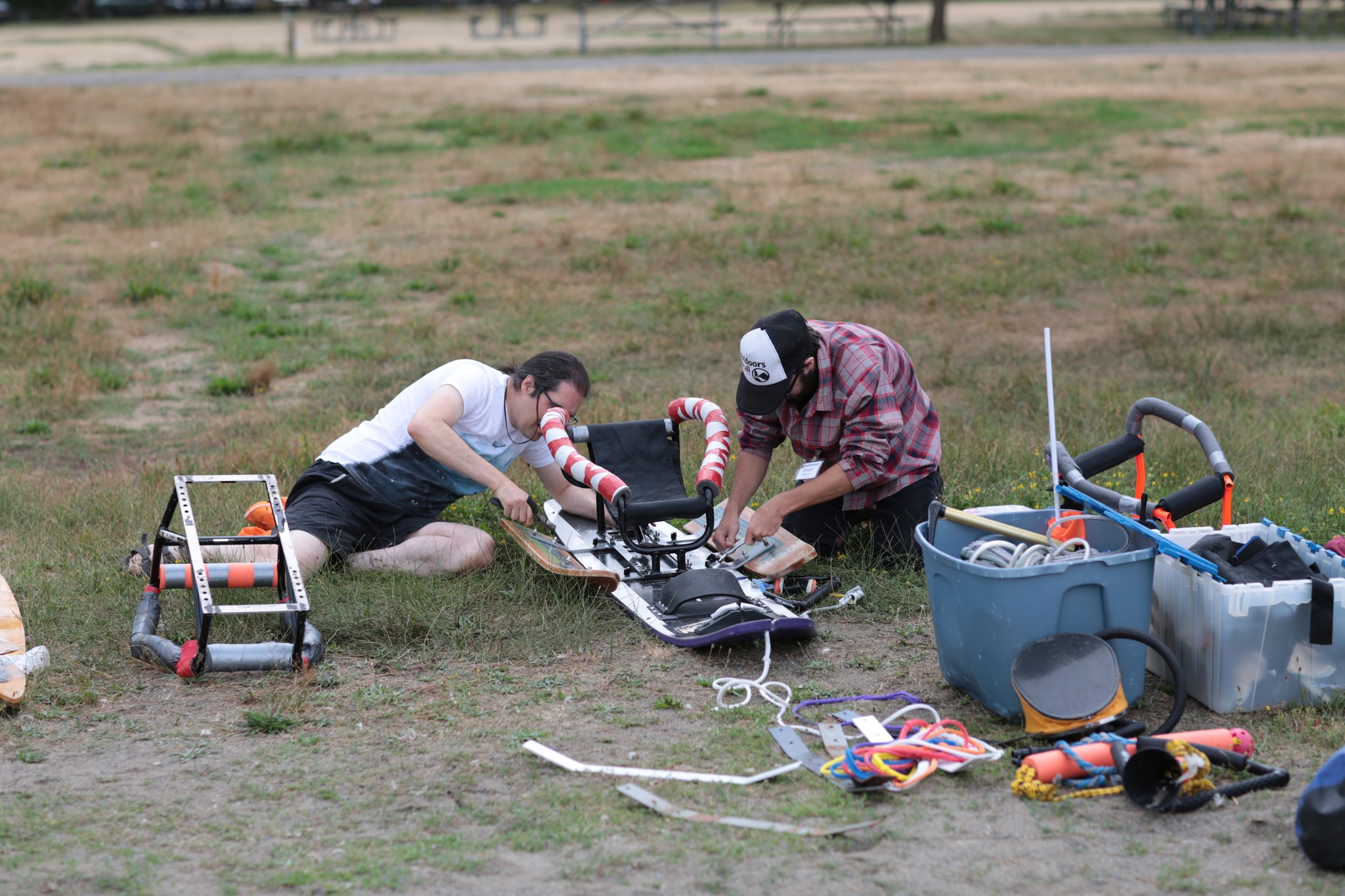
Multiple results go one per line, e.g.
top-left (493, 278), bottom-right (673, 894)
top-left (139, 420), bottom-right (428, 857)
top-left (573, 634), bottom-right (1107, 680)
top-left (539, 383), bottom-right (578, 426)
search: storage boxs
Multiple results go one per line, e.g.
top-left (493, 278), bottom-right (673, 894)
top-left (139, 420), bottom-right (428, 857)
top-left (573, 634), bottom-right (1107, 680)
top-left (914, 504), bottom-right (1160, 725)
top-left (1146, 517), bottom-right (1345, 716)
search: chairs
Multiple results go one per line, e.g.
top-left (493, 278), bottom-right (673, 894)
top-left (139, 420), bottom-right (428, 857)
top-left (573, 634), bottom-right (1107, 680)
top-left (541, 396), bottom-right (729, 584)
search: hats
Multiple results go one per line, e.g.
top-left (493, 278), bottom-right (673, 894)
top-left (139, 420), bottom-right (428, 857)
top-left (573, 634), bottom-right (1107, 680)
top-left (736, 309), bottom-right (811, 416)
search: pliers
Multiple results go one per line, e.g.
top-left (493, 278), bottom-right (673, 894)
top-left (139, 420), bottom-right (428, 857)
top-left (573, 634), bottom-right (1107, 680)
top-left (489, 494), bottom-right (558, 531)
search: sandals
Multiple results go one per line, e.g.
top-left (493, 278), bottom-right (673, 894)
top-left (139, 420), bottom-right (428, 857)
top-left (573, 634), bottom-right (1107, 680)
top-left (122, 532), bottom-right (189, 581)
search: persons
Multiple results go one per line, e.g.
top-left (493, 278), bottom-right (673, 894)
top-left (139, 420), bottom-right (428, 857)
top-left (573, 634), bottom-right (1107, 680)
top-left (712, 309), bottom-right (943, 579)
top-left (124, 352), bottom-right (657, 586)
